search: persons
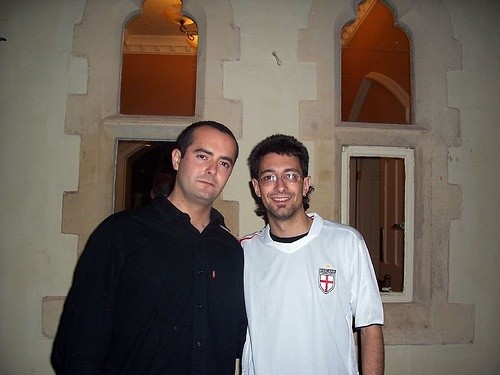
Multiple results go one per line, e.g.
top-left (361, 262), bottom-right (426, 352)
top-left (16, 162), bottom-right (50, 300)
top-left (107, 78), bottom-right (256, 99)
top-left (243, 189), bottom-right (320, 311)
top-left (67, 120), bottom-right (249, 375)
top-left (237, 133), bottom-right (385, 375)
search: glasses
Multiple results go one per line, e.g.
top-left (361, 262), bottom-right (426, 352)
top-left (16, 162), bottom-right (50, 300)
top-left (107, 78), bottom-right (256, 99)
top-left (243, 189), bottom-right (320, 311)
top-left (256, 173), bottom-right (304, 187)
